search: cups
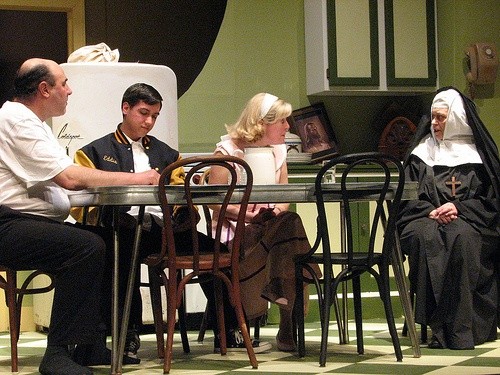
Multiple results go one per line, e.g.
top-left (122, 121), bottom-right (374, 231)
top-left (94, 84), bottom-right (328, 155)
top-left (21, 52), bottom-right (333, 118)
top-left (322, 159), bottom-right (336, 184)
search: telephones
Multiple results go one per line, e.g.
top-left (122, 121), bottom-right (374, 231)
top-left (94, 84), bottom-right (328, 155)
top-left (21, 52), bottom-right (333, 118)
top-left (465, 42), bottom-right (497, 85)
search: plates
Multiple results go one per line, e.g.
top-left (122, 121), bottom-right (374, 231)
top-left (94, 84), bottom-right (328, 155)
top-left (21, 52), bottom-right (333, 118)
top-left (286, 153), bottom-right (312, 165)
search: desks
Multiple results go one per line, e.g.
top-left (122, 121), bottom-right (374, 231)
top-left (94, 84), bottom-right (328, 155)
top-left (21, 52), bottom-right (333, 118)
top-left (67, 176), bottom-right (421, 375)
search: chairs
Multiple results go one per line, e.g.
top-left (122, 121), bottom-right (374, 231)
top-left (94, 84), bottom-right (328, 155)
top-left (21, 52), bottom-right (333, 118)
top-left (0, 142), bottom-right (424, 375)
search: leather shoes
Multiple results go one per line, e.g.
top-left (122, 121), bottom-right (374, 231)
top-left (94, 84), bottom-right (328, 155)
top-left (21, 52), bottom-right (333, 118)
top-left (276, 330), bottom-right (297, 352)
top-left (260, 288), bottom-right (287, 305)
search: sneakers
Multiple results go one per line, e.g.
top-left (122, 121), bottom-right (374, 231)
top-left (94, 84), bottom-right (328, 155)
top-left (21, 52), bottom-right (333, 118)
top-left (214, 328), bottom-right (272, 352)
top-left (123, 325), bottom-right (140, 353)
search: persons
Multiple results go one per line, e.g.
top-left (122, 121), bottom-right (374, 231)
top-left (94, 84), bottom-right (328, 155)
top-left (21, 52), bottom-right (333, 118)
top-left (393, 85), bottom-right (500, 348)
top-left (72, 83), bottom-right (271, 357)
top-left (208, 93), bottom-right (323, 351)
top-left (0, 58), bottom-right (141, 375)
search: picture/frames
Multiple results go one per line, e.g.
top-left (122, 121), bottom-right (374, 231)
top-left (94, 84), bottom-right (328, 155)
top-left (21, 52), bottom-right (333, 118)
top-left (288, 102), bottom-right (341, 163)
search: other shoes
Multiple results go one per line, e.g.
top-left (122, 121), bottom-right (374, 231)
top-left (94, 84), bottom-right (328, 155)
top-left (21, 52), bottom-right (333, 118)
top-left (73, 349), bottom-right (138, 366)
top-left (40, 353), bottom-right (93, 375)
top-left (427, 335), bottom-right (440, 349)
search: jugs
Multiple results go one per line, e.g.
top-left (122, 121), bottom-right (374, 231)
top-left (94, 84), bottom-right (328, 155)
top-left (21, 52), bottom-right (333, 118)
top-left (232, 145), bottom-right (277, 184)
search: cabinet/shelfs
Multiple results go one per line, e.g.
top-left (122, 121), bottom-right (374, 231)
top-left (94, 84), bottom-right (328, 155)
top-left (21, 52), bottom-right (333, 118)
top-left (265, 172), bottom-right (421, 324)
top-left (304, 0), bottom-right (440, 98)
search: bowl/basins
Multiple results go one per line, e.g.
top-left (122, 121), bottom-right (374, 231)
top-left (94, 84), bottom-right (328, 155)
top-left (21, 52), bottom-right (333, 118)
top-left (284, 131), bottom-right (305, 152)
